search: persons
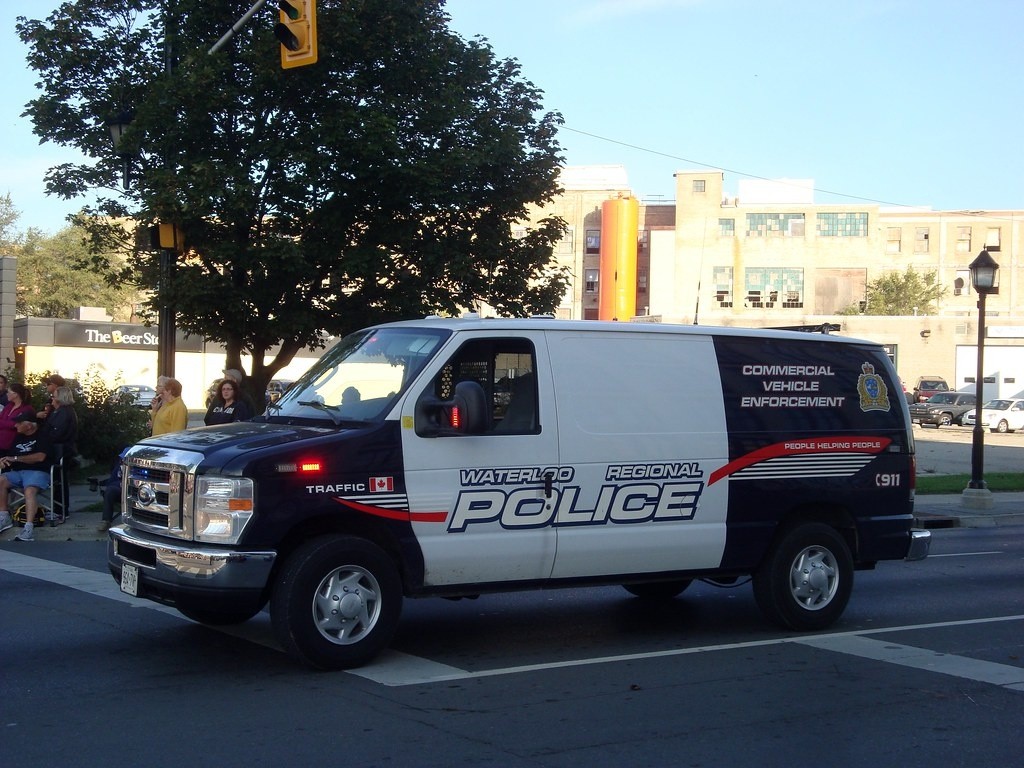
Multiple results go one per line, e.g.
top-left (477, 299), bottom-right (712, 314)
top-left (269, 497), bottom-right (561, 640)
top-left (202, 368), bottom-right (253, 420)
top-left (0, 383), bottom-right (36, 474)
top-left (150, 379), bottom-right (188, 436)
top-left (98, 446), bottom-right (132, 531)
top-left (205, 380), bottom-right (245, 426)
top-left (0, 411), bottom-right (54, 542)
top-left (341, 386), bottom-right (360, 404)
top-left (148, 375), bottom-right (170, 429)
top-left (0, 375), bottom-right (8, 412)
top-left (44, 386), bottom-right (77, 521)
top-left (36, 375), bottom-right (65, 418)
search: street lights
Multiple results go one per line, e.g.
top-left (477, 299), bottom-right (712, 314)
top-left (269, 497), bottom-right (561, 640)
top-left (965, 249), bottom-right (1001, 499)
top-left (104, 105), bottom-right (189, 435)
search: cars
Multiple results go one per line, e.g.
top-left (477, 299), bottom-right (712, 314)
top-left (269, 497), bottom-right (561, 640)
top-left (962, 398), bottom-right (1024, 436)
top-left (111, 385), bottom-right (157, 410)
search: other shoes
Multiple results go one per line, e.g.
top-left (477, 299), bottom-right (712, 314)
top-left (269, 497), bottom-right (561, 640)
top-left (95, 520), bottom-right (112, 531)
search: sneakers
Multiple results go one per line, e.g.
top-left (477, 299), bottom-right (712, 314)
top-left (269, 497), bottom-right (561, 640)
top-left (0, 512), bottom-right (14, 532)
top-left (14, 528), bottom-right (35, 541)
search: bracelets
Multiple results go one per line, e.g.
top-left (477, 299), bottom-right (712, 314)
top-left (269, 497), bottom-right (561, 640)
top-left (14, 456), bottom-right (17, 461)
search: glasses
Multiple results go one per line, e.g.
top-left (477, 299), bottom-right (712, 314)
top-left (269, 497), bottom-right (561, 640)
top-left (5, 389), bottom-right (16, 394)
top-left (46, 381), bottom-right (57, 387)
top-left (221, 387), bottom-right (234, 391)
top-left (0, 381), bottom-right (4, 384)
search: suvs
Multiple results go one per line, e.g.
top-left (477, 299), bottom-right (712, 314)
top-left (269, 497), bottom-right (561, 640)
top-left (913, 375), bottom-right (955, 402)
top-left (909, 392), bottom-right (981, 429)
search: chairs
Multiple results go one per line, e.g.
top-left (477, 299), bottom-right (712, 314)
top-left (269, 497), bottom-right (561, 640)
top-left (1, 443), bottom-right (65, 527)
top-left (87, 473), bottom-right (123, 526)
top-left (495, 371), bottom-right (538, 432)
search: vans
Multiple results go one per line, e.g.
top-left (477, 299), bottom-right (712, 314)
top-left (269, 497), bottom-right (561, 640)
top-left (105, 317), bottom-right (932, 677)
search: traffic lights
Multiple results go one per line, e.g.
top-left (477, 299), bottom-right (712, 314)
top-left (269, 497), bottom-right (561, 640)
top-left (275, 0), bottom-right (321, 72)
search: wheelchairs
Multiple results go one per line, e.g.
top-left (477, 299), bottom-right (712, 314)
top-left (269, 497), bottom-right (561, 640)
top-left (5, 454), bottom-right (65, 527)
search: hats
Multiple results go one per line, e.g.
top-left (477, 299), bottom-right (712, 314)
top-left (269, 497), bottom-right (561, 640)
top-left (222, 369), bottom-right (242, 383)
top-left (119, 447), bottom-right (131, 458)
top-left (41, 374), bottom-right (65, 386)
top-left (10, 408), bottom-right (37, 422)
